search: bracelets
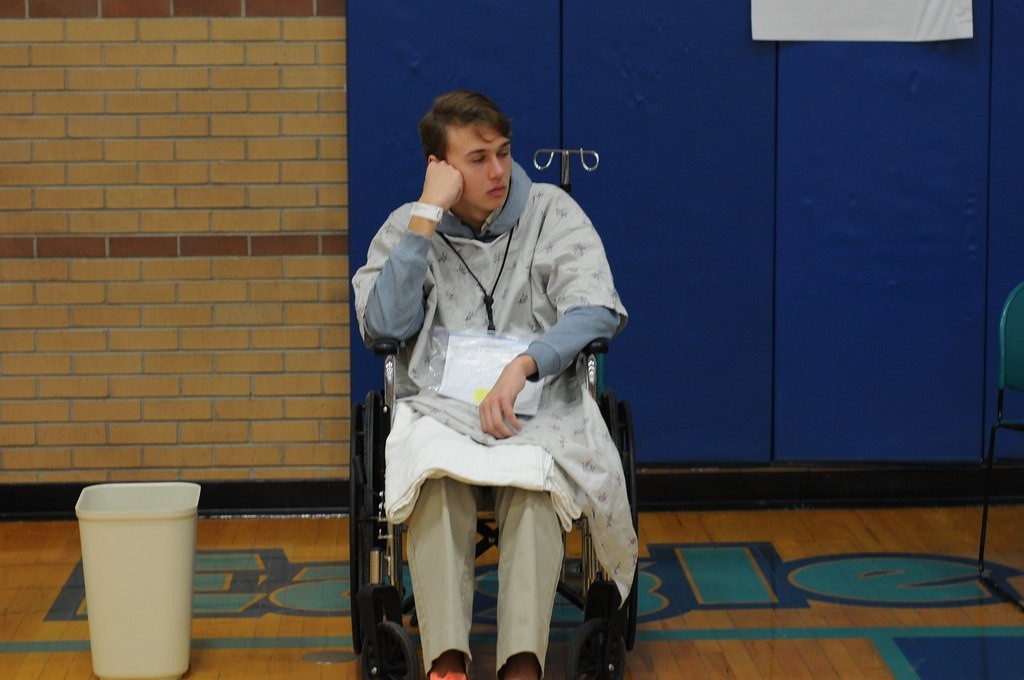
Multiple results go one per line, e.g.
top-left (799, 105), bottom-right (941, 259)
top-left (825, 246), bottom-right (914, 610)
top-left (411, 203), bottom-right (444, 224)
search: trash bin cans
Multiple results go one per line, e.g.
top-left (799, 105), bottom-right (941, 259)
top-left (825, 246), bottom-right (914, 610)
top-left (74, 481), bottom-right (202, 680)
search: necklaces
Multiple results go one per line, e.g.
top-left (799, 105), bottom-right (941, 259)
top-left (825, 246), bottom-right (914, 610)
top-left (435, 227), bottom-right (513, 331)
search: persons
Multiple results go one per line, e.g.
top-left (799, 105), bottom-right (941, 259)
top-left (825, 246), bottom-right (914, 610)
top-left (353, 91), bottom-right (630, 680)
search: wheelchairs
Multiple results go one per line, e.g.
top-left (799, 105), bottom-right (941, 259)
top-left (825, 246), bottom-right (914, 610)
top-left (347, 333), bottom-right (643, 679)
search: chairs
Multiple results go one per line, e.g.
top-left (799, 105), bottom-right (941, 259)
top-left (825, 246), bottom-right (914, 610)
top-left (978, 284), bottom-right (1024, 572)
